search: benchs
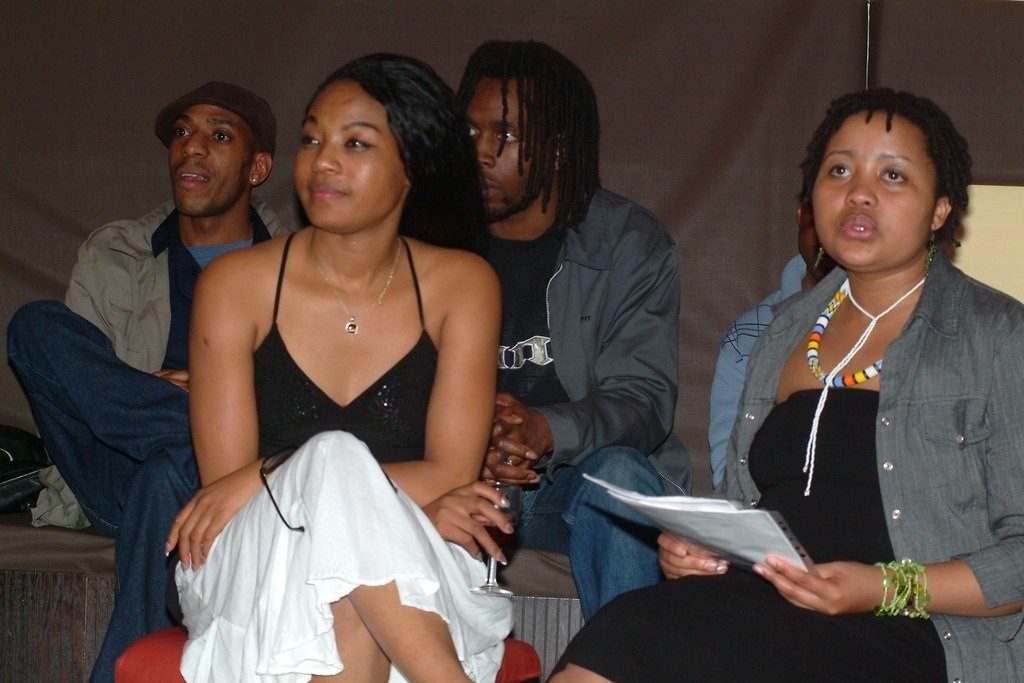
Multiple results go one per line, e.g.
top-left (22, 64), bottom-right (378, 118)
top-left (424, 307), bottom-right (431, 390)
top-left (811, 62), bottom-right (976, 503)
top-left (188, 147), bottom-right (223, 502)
top-left (0, 519), bottom-right (587, 683)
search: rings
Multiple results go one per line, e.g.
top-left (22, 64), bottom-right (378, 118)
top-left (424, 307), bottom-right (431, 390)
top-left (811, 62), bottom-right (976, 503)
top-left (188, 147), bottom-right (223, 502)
top-left (504, 458), bottom-right (513, 467)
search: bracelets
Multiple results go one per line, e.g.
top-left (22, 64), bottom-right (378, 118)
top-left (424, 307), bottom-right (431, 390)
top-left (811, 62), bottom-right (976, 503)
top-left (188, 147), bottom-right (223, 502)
top-left (875, 561), bottom-right (930, 621)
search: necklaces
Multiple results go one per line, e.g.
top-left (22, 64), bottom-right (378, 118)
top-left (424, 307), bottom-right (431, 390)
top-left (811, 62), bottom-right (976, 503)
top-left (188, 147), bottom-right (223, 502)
top-left (802, 279), bottom-right (925, 497)
top-left (309, 237), bottom-right (402, 335)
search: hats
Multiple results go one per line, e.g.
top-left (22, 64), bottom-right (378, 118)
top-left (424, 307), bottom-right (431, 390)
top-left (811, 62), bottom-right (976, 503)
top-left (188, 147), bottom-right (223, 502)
top-left (155, 82), bottom-right (277, 158)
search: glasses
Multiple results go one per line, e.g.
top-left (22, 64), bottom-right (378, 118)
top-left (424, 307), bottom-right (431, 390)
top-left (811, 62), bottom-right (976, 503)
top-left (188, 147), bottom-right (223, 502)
top-left (260, 445), bottom-right (397, 532)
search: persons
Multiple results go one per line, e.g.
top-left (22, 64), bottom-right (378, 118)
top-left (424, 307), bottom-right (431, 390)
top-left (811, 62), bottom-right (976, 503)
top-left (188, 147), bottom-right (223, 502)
top-left (7, 40), bottom-right (681, 683)
top-left (547, 89), bottom-right (1024, 683)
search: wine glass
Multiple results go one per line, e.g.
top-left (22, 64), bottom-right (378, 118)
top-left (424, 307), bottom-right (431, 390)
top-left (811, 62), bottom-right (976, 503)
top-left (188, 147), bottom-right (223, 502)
top-left (470, 467), bottom-right (525, 598)
